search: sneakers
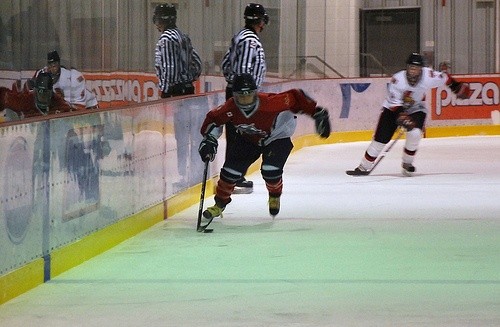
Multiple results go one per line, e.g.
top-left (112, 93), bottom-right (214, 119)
top-left (269, 194), bottom-right (280, 219)
top-left (402, 162), bottom-right (415, 176)
top-left (355, 164), bottom-right (367, 173)
top-left (231, 177), bottom-right (253, 193)
top-left (202, 202), bottom-right (225, 222)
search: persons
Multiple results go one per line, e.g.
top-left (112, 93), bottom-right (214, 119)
top-left (0, 53), bottom-right (104, 214)
top-left (222, 3), bottom-right (271, 195)
top-left (148, 2), bottom-right (205, 187)
top-left (199, 73), bottom-right (331, 222)
top-left (347, 53), bottom-right (469, 177)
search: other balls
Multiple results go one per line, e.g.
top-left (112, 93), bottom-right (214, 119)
top-left (204, 228), bottom-right (214, 233)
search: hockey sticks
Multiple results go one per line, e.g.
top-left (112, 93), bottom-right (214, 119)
top-left (346, 125), bottom-right (410, 176)
top-left (196, 153), bottom-right (214, 233)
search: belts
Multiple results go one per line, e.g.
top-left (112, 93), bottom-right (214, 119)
top-left (185, 84), bottom-right (192, 87)
top-left (227, 83), bottom-right (234, 88)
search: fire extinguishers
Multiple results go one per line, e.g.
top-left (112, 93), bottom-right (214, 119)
top-left (439, 60), bottom-right (450, 71)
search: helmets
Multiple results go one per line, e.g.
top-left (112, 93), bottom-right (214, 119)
top-left (243, 3), bottom-right (269, 24)
top-left (34, 71), bottom-right (54, 118)
top-left (153, 3), bottom-right (177, 24)
top-left (47, 50), bottom-right (60, 65)
top-left (232, 74), bottom-right (257, 112)
top-left (406, 52), bottom-right (424, 67)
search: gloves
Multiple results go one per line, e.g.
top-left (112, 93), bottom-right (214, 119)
top-left (449, 81), bottom-right (463, 93)
top-left (311, 106), bottom-right (330, 139)
top-left (397, 113), bottom-right (416, 132)
top-left (198, 134), bottom-right (218, 161)
